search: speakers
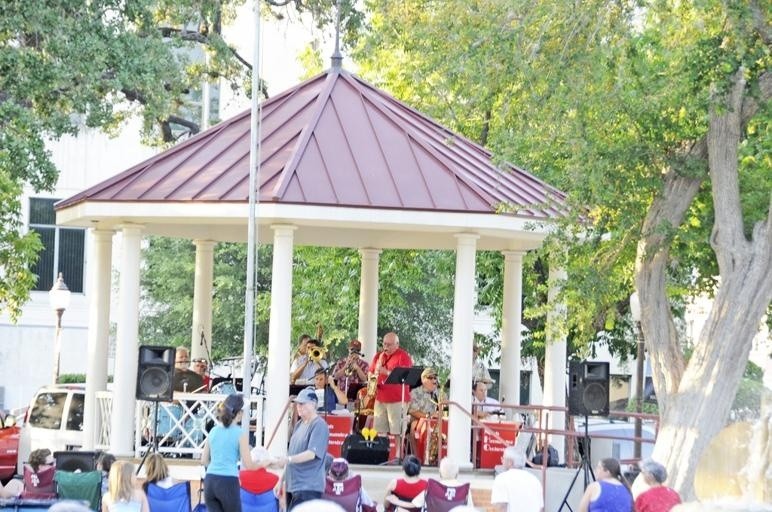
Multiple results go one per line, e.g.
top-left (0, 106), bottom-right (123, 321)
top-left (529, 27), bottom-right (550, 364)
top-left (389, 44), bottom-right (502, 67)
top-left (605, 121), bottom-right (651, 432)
top-left (135, 345), bottom-right (176, 402)
top-left (340, 434), bottom-right (390, 464)
top-left (55, 452), bottom-right (98, 472)
top-left (569, 360), bottom-right (611, 415)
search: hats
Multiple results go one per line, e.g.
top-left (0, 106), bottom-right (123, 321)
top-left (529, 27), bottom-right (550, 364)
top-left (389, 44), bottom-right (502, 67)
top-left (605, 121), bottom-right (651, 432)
top-left (349, 340), bottom-right (361, 350)
top-left (291, 389), bottom-right (318, 404)
top-left (421, 368), bottom-right (438, 379)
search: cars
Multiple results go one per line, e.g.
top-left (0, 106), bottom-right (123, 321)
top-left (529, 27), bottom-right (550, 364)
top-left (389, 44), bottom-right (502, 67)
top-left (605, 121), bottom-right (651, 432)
top-left (0, 406), bottom-right (29, 484)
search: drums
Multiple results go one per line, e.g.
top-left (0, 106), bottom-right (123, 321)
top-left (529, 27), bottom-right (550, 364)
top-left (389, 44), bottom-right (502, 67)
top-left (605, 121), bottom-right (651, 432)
top-left (148, 404), bottom-right (185, 443)
top-left (211, 381), bottom-right (237, 395)
top-left (183, 411), bottom-right (220, 448)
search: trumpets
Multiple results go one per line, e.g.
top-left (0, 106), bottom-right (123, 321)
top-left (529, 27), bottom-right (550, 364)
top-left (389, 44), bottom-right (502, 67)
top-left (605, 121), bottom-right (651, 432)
top-left (308, 346), bottom-right (329, 361)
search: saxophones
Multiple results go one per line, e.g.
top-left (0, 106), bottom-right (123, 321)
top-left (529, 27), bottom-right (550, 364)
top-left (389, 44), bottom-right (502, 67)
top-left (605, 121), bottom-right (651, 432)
top-left (344, 355), bottom-right (359, 377)
top-left (368, 350), bottom-right (388, 398)
top-left (425, 383), bottom-right (444, 465)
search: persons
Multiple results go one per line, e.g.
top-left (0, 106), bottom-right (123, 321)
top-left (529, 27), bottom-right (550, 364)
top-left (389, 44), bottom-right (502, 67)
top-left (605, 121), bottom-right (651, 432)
top-left (141, 453), bottom-right (178, 495)
top-left (95, 453), bottom-right (117, 496)
top-left (576, 456), bottom-right (635, 512)
top-left (326, 457), bottom-right (377, 511)
top-left (438, 455), bottom-right (475, 511)
top-left (173, 346), bottom-right (205, 393)
top-left (192, 357), bottom-right (214, 394)
top-left (490, 449), bottom-right (545, 512)
top-left (199, 394), bottom-right (278, 512)
top-left (290, 331), bottom-right (506, 466)
top-left (28, 448), bottom-right (57, 474)
top-left (384, 454), bottom-right (428, 511)
top-left (270, 384), bottom-right (333, 512)
top-left (100, 459), bottom-right (150, 512)
top-left (631, 460), bottom-right (682, 512)
top-left (248, 444), bottom-right (272, 470)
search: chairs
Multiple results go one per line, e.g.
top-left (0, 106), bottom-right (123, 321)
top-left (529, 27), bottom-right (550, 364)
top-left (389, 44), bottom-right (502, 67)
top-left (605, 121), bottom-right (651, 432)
top-left (0, 466), bottom-right (208, 512)
top-left (321, 474), bottom-right (364, 512)
top-left (424, 477), bottom-right (470, 512)
top-left (238, 485), bottom-right (278, 512)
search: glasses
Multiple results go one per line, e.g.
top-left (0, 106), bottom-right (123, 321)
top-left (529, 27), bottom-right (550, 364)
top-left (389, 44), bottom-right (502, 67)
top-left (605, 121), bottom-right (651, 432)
top-left (45, 458), bottom-right (56, 465)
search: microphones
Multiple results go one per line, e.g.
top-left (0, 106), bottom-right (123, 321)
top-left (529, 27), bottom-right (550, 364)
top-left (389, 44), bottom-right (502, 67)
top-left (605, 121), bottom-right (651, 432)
top-left (199, 327), bottom-right (205, 347)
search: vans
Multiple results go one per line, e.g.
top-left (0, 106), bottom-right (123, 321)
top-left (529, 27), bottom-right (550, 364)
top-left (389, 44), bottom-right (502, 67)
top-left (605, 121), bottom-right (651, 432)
top-left (17, 383), bottom-right (205, 483)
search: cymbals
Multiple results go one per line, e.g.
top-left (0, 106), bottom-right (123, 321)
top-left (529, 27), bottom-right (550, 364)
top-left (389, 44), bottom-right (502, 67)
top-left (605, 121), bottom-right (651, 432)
top-left (173, 370), bottom-right (204, 397)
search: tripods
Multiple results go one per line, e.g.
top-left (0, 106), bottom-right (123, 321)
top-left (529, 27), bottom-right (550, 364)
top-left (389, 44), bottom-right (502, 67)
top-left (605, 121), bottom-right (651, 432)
top-left (559, 420), bottom-right (596, 512)
top-left (132, 404), bottom-right (160, 478)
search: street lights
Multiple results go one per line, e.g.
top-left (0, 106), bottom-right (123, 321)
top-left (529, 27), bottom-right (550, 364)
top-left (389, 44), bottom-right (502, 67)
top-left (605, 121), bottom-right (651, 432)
top-left (48, 271), bottom-right (71, 383)
top-left (629, 290), bottom-right (644, 471)
